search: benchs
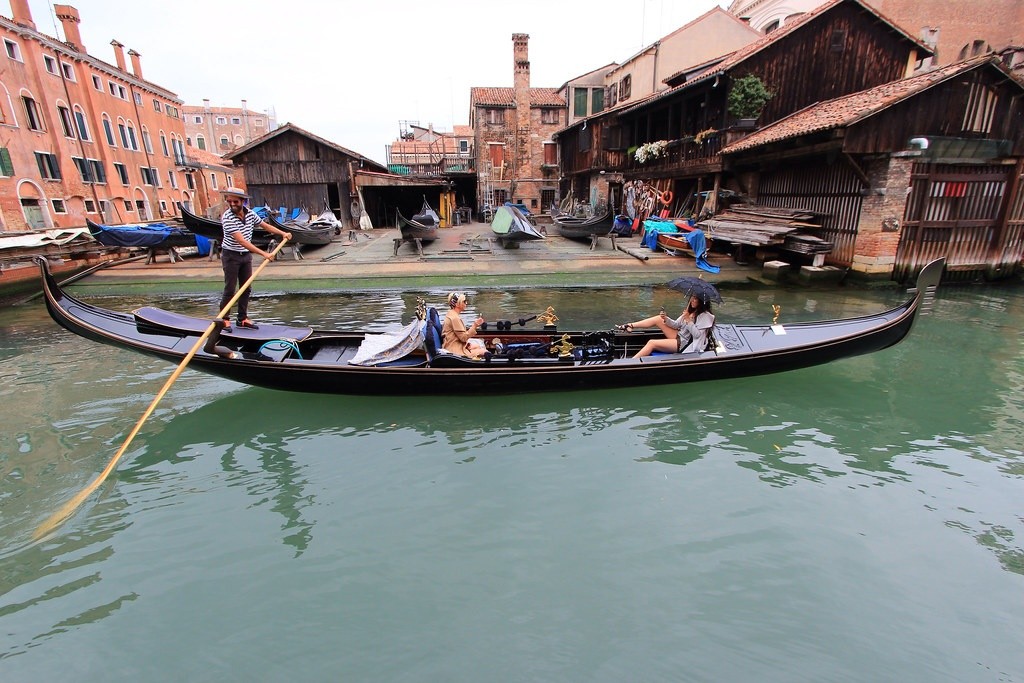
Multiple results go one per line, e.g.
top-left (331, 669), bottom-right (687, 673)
top-left (423, 306), bottom-right (466, 366)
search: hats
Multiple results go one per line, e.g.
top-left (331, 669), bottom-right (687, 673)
top-left (219, 187), bottom-right (253, 198)
top-left (692, 293), bottom-right (711, 302)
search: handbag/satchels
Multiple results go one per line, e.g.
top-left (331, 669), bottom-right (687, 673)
top-left (705, 328), bottom-right (718, 356)
top-left (463, 338), bottom-right (489, 360)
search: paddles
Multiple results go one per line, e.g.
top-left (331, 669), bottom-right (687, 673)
top-left (94, 237), bottom-right (288, 480)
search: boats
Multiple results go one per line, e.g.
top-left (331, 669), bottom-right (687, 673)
top-left (490, 203), bottom-right (545, 241)
top-left (84, 216), bottom-right (201, 250)
top-left (550, 186), bottom-right (616, 238)
top-left (265, 197), bottom-right (341, 245)
top-left (395, 194), bottom-right (441, 242)
top-left (641, 223), bottom-right (713, 255)
top-left (615, 214), bottom-right (633, 237)
top-left (29, 251), bottom-right (950, 400)
top-left (177, 201), bottom-right (311, 240)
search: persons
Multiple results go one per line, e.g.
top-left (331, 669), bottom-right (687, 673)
top-left (442, 293), bottom-right (491, 358)
top-left (614, 293), bottom-right (715, 359)
top-left (220, 188), bottom-right (292, 329)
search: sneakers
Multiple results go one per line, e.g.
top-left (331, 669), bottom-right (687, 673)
top-left (223, 319), bottom-right (232, 332)
top-left (235, 318), bottom-right (259, 330)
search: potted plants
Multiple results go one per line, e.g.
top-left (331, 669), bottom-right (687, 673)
top-left (727, 72), bottom-right (778, 127)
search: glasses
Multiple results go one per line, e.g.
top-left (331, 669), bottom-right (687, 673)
top-left (227, 199), bottom-right (240, 204)
top-left (462, 300), bottom-right (468, 304)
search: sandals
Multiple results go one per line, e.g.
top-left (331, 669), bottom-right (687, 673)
top-left (614, 323), bottom-right (634, 333)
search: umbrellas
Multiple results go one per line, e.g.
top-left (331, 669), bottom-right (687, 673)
top-left (662, 274), bottom-right (724, 323)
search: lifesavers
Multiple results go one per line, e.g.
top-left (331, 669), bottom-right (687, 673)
top-left (660, 190), bottom-right (673, 205)
